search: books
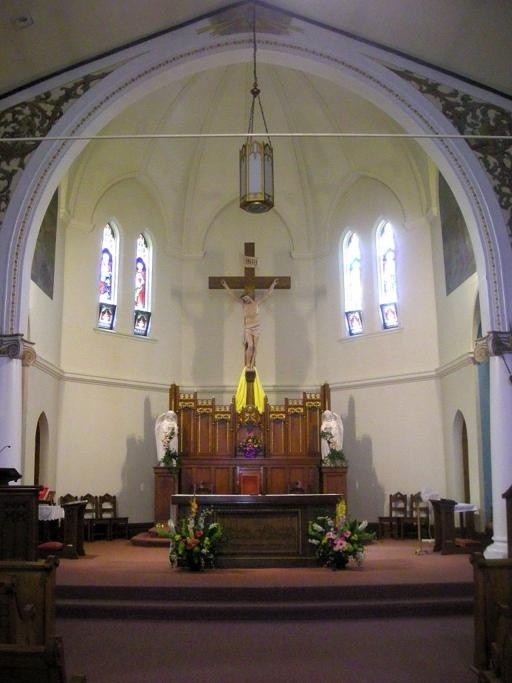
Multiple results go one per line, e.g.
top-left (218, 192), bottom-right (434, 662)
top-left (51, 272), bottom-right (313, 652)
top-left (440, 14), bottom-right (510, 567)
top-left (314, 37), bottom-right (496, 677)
top-left (39, 486), bottom-right (51, 500)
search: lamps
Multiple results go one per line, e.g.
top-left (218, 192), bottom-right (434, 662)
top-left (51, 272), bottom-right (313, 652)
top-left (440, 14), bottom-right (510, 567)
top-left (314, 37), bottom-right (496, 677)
top-left (240, 0), bottom-right (274, 213)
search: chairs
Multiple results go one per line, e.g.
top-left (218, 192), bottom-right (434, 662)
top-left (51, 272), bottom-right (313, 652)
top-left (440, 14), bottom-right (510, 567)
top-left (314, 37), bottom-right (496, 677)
top-left (80, 493), bottom-right (110, 542)
top-left (400, 491), bottom-right (430, 542)
top-left (99, 493), bottom-right (129, 540)
top-left (378, 492), bottom-right (407, 539)
top-left (59, 494), bottom-right (78, 504)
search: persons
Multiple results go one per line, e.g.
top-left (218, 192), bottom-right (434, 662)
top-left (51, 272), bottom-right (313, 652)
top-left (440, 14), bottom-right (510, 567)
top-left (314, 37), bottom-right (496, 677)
top-left (319, 409), bottom-right (341, 464)
top-left (99, 248), bottom-right (113, 298)
top-left (135, 257), bottom-right (147, 307)
top-left (155, 410), bottom-right (179, 468)
top-left (220, 277), bottom-right (279, 370)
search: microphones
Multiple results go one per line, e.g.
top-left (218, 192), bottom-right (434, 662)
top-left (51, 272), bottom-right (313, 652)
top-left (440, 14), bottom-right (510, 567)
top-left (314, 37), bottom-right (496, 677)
top-left (0, 446), bottom-right (11, 453)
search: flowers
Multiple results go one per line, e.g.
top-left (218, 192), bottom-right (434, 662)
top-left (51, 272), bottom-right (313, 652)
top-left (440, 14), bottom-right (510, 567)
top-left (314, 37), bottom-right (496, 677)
top-left (306, 497), bottom-right (376, 569)
top-left (154, 492), bottom-right (224, 572)
top-left (239, 436), bottom-right (263, 457)
top-left (319, 428), bottom-right (348, 467)
top-left (160, 427), bottom-right (179, 466)
